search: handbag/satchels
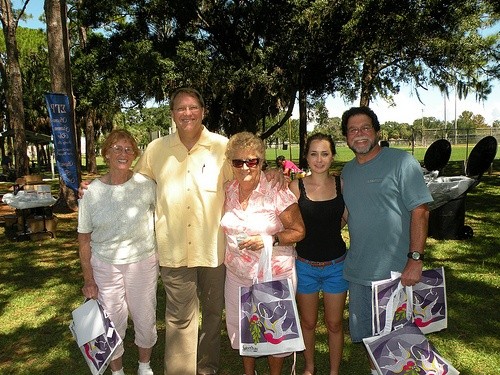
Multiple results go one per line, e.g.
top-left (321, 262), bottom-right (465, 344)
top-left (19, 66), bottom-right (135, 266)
top-left (361, 281), bottom-right (461, 375)
top-left (238, 234), bottom-right (307, 356)
top-left (371, 265), bottom-right (447, 336)
top-left (68, 296), bottom-right (125, 375)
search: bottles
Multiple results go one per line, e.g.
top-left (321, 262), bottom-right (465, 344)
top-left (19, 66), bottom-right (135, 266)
top-left (290, 169), bottom-right (311, 181)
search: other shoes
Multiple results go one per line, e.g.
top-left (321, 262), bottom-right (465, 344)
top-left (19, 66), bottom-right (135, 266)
top-left (137, 362), bottom-right (154, 375)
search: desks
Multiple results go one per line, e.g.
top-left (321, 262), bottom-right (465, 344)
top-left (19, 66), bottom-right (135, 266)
top-left (15, 201), bottom-right (55, 241)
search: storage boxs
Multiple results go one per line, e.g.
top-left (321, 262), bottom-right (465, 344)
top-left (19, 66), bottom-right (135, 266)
top-left (16, 173), bottom-right (53, 200)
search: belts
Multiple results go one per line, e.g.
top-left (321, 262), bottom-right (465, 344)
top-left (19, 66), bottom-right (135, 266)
top-left (296, 253), bottom-right (347, 267)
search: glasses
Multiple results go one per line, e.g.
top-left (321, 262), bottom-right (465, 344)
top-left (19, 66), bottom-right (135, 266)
top-left (169, 105), bottom-right (202, 112)
top-left (109, 145), bottom-right (137, 155)
top-left (346, 125), bottom-right (374, 134)
top-left (232, 157), bottom-right (260, 169)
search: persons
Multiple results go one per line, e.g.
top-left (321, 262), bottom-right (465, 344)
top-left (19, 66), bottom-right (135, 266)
top-left (78, 128), bottom-right (159, 375)
top-left (341, 106), bottom-right (434, 375)
top-left (275, 155), bottom-right (304, 180)
top-left (78, 88), bottom-right (289, 375)
top-left (221, 132), bottom-right (306, 375)
top-left (288, 132), bottom-right (349, 375)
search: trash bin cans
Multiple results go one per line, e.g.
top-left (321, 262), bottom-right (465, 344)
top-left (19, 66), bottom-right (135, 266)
top-left (422, 175), bottom-right (474, 241)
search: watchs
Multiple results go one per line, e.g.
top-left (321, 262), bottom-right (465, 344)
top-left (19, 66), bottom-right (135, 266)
top-left (272, 234), bottom-right (279, 246)
top-left (407, 252), bottom-right (425, 262)
